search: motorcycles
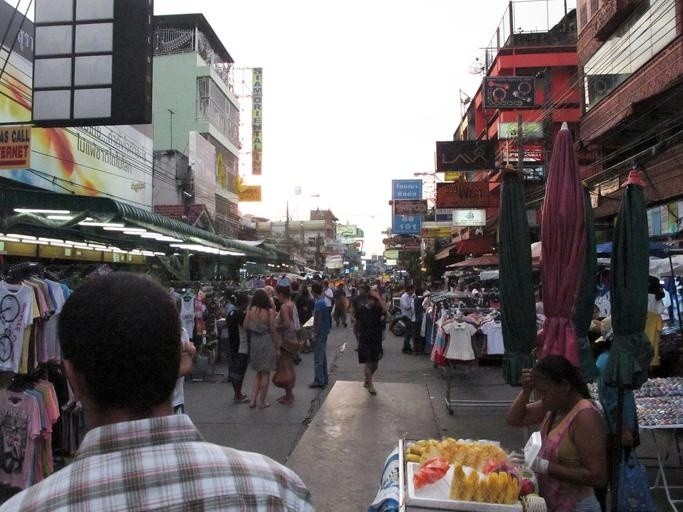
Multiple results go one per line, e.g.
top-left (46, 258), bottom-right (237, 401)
top-left (389, 304), bottom-right (407, 337)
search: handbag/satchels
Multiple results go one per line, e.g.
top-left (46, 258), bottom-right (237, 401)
top-left (272, 358), bottom-right (289, 387)
top-left (281, 338), bottom-right (298, 354)
top-left (605, 447), bottom-right (655, 512)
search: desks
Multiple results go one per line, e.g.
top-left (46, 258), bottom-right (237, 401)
top-left (638, 421), bottom-right (683, 511)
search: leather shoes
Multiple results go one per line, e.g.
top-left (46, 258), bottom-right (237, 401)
top-left (233, 394), bottom-right (294, 408)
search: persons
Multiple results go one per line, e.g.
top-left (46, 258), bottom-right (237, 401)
top-left (593, 314), bottom-right (657, 512)
top-left (217, 261), bottom-right (429, 410)
top-left (0, 271), bottom-right (322, 511)
top-left (504, 355), bottom-right (610, 512)
top-left (641, 275), bottom-right (664, 382)
top-left (171, 298), bottom-right (197, 417)
top-left (592, 304), bottom-right (605, 321)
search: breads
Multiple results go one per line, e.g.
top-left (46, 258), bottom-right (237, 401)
top-left (402, 438), bottom-right (522, 503)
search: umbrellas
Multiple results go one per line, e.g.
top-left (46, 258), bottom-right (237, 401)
top-left (575, 174), bottom-right (600, 390)
top-left (536, 116), bottom-right (586, 375)
top-left (495, 160), bottom-right (535, 443)
top-left (603, 163), bottom-right (652, 512)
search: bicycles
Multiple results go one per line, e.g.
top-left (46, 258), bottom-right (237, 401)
top-left (205, 315), bottom-right (227, 368)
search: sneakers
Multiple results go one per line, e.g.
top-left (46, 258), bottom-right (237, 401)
top-left (369, 384), bottom-right (376, 394)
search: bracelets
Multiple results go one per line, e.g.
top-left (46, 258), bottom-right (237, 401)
top-left (535, 457), bottom-right (549, 476)
